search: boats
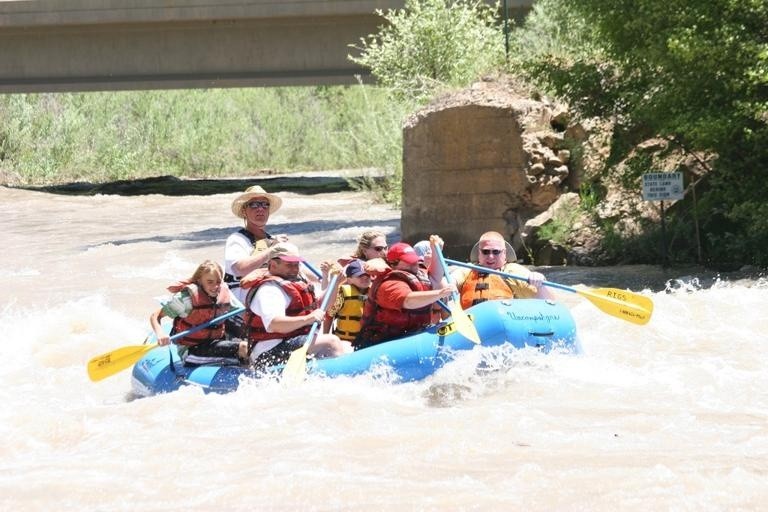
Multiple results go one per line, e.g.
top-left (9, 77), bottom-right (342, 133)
top-left (129, 281), bottom-right (578, 400)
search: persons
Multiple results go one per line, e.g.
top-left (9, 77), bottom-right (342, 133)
top-left (414, 240), bottom-right (452, 326)
top-left (332, 258), bottom-right (371, 342)
top-left (222, 185), bottom-right (288, 307)
top-left (430, 231), bottom-right (556, 311)
top-left (321, 231), bottom-right (389, 291)
top-left (244, 242), bottom-right (345, 367)
top-left (350, 242), bottom-right (458, 353)
top-left (150, 259), bottom-right (253, 369)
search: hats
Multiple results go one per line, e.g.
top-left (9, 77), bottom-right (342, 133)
top-left (346, 260), bottom-right (370, 277)
top-left (231, 186), bottom-right (282, 218)
top-left (386, 243), bottom-right (424, 268)
top-left (270, 243), bottom-right (304, 262)
top-left (470, 230), bottom-right (517, 263)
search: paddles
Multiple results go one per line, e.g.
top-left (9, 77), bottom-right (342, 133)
top-left (443, 257), bottom-right (653, 324)
top-left (278, 271), bottom-right (339, 382)
top-left (88, 307), bottom-right (246, 383)
top-left (430, 235), bottom-right (481, 344)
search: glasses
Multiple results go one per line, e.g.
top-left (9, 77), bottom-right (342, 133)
top-left (370, 246), bottom-right (387, 251)
top-left (481, 249), bottom-right (503, 255)
top-left (243, 201), bottom-right (270, 208)
